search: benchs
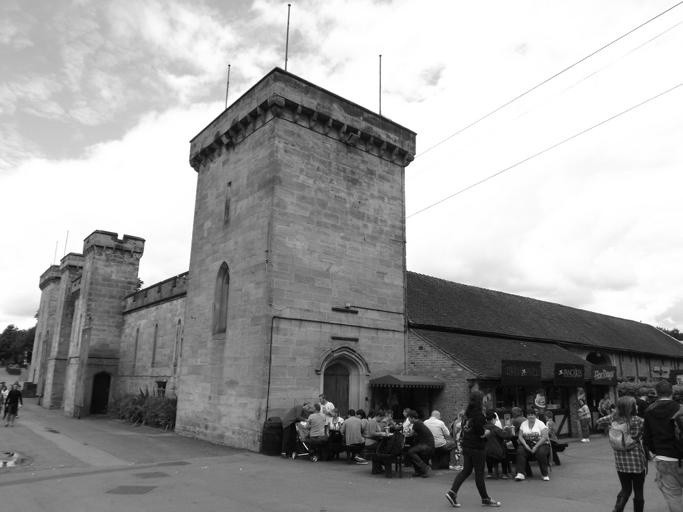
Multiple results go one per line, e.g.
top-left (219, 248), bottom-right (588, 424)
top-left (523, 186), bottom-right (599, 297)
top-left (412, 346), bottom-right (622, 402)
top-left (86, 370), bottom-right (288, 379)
top-left (551, 443), bottom-right (568, 465)
top-left (508, 452), bottom-right (535, 461)
top-left (413, 451), bottom-right (431, 473)
top-left (340, 444), bottom-right (361, 464)
top-left (432, 450), bottom-right (453, 470)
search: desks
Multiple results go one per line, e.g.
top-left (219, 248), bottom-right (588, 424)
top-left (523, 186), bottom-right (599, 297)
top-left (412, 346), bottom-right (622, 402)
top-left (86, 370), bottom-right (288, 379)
top-left (329, 427), bottom-right (339, 431)
top-left (363, 431), bottom-right (410, 478)
top-left (511, 433), bottom-right (533, 478)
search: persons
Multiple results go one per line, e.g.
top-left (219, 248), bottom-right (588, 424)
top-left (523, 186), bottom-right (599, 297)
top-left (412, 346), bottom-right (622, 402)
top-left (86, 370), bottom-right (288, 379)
top-left (598, 379), bottom-right (683, 512)
top-left (578, 399), bottom-right (592, 443)
top-left (281, 391), bottom-right (561, 507)
top-left (0, 381), bottom-right (23, 427)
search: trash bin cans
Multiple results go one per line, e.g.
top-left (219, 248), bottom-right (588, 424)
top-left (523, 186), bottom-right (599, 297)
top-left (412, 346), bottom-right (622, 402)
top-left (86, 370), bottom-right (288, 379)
top-left (21, 382), bottom-right (37, 399)
top-left (262, 416), bottom-right (283, 457)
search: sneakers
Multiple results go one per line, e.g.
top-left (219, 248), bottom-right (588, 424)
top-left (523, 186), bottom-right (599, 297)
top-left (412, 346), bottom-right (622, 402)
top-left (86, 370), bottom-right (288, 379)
top-left (541, 476), bottom-right (549, 481)
top-left (481, 498), bottom-right (500, 507)
top-left (354, 455), bottom-right (365, 462)
top-left (355, 462), bottom-right (368, 465)
top-left (445, 492), bottom-right (460, 508)
top-left (514, 473), bottom-right (525, 481)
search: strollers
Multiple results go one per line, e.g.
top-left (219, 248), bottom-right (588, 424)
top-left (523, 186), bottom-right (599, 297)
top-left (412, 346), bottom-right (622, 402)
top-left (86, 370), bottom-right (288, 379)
top-left (279, 420), bottom-right (320, 464)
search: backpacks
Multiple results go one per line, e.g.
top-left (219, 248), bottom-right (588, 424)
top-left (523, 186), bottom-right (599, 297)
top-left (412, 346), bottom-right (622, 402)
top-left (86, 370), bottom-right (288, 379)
top-left (608, 421), bottom-right (638, 453)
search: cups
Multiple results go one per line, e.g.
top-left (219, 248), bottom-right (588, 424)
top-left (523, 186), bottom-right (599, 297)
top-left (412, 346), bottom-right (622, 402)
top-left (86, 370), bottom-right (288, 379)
top-left (385, 426), bottom-right (389, 434)
top-left (510, 426), bottom-right (515, 435)
